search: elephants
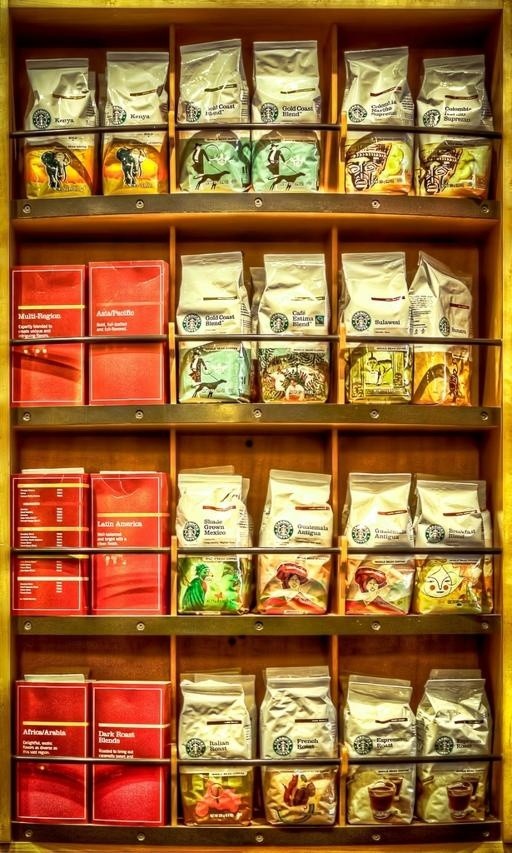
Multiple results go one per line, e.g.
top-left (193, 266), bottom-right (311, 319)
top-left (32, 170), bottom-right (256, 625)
top-left (40, 151), bottom-right (71, 191)
top-left (116, 145), bottom-right (147, 188)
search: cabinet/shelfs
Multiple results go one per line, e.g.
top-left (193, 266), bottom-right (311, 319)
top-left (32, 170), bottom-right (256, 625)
top-left (2, 3), bottom-right (506, 846)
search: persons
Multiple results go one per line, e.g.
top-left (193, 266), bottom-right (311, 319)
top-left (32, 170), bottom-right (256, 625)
top-left (189, 141), bottom-right (211, 175)
top-left (267, 143), bottom-right (285, 175)
top-left (270, 364), bottom-right (286, 399)
top-left (286, 572), bottom-right (302, 589)
top-left (263, 370), bottom-right (271, 383)
top-left (189, 351), bottom-right (207, 382)
top-left (364, 578), bottom-right (378, 594)
top-left (287, 378), bottom-right (305, 399)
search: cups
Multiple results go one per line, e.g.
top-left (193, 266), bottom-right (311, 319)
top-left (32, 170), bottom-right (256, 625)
top-left (368, 785), bottom-right (394, 820)
top-left (461, 775), bottom-right (480, 801)
top-left (383, 775), bottom-right (402, 800)
top-left (447, 782), bottom-right (473, 818)
